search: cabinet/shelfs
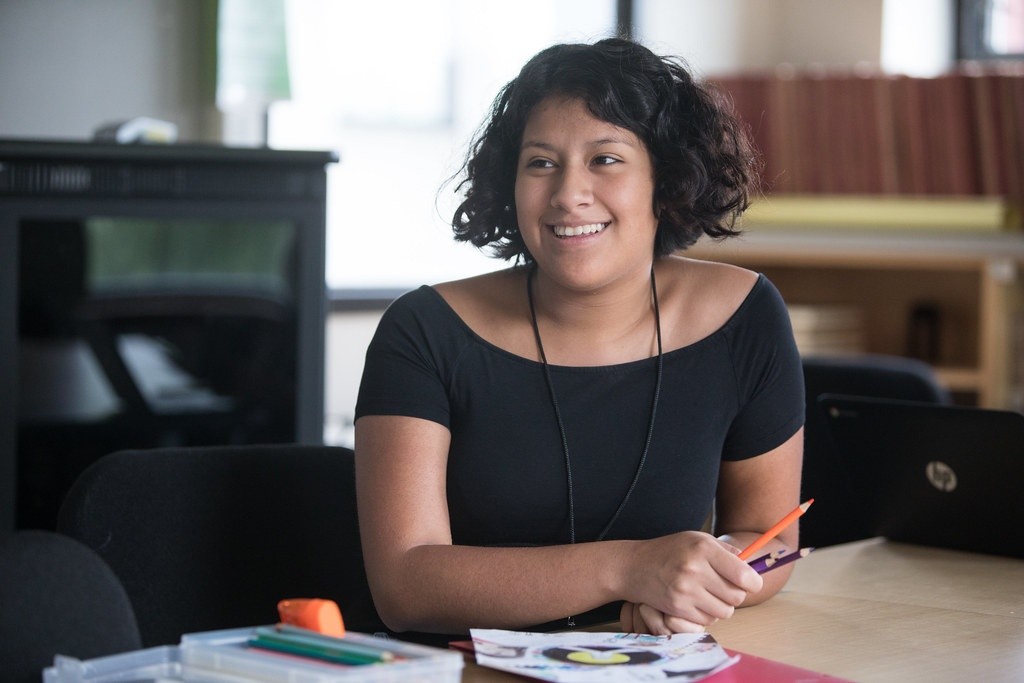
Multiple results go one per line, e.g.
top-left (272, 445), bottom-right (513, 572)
top-left (0, 135), bottom-right (342, 528)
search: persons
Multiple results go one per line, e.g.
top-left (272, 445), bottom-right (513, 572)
top-left (354, 38), bottom-right (805, 636)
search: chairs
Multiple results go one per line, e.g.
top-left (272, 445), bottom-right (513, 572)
top-left (0, 532), bottom-right (142, 683)
top-left (797, 353), bottom-right (950, 550)
top-left (55, 447), bottom-right (383, 650)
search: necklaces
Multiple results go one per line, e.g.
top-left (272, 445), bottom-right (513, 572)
top-left (527, 265), bottom-right (663, 628)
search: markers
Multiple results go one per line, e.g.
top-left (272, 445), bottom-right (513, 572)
top-left (747, 548), bottom-right (815, 574)
top-left (737, 498), bottom-right (814, 561)
top-left (247, 622), bottom-right (395, 665)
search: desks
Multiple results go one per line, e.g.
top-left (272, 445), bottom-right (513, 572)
top-left (447, 535), bottom-right (1024, 683)
